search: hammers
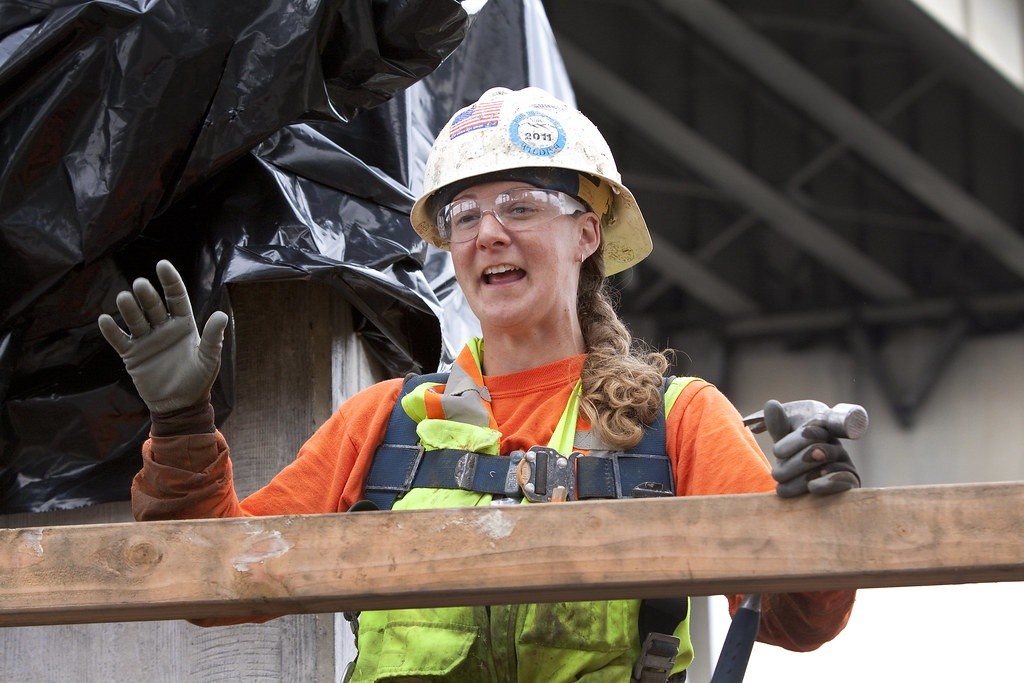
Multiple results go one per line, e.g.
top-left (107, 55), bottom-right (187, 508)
top-left (709, 398), bottom-right (871, 683)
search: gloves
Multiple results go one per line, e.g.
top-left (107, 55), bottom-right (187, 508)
top-left (763, 400), bottom-right (861, 500)
top-left (97, 261), bottom-right (229, 437)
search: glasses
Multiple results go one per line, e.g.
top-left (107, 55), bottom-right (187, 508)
top-left (436, 189), bottom-right (588, 244)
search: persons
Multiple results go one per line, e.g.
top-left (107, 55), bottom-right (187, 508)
top-left (98, 87), bottom-right (862, 683)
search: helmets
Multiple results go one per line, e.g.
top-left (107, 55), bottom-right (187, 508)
top-left (409, 85), bottom-right (653, 278)
